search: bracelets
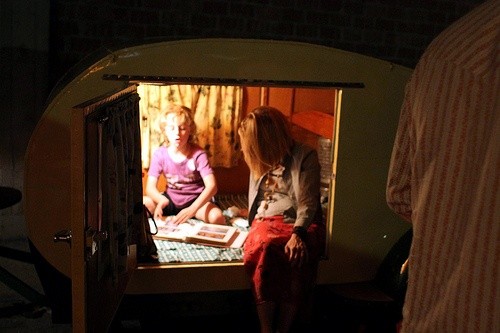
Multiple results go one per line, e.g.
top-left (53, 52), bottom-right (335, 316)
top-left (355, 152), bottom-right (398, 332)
top-left (292, 226), bottom-right (308, 239)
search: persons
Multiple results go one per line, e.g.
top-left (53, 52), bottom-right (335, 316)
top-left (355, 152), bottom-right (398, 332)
top-left (138, 102), bottom-right (229, 230)
top-left (237, 104), bottom-right (323, 333)
top-left (386, 0), bottom-right (500, 333)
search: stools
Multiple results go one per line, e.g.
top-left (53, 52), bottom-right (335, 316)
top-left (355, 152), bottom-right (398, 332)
top-left (0, 186), bottom-right (46, 309)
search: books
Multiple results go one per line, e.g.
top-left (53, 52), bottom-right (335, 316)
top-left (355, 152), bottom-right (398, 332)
top-left (148, 214), bottom-right (240, 249)
